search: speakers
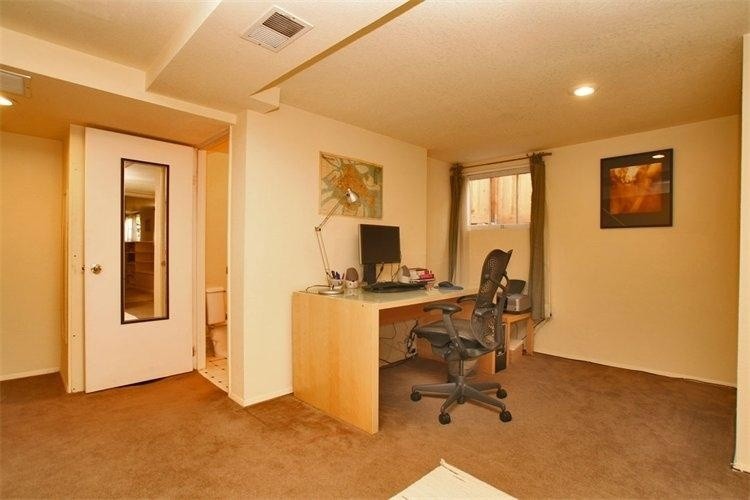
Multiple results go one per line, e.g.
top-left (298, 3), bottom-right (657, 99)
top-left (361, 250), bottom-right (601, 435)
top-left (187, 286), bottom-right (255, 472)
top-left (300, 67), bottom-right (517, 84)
top-left (345, 267), bottom-right (359, 289)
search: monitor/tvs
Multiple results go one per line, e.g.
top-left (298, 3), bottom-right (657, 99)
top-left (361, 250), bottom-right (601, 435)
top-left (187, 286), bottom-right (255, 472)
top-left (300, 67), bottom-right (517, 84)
top-left (360, 224), bottom-right (401, 284)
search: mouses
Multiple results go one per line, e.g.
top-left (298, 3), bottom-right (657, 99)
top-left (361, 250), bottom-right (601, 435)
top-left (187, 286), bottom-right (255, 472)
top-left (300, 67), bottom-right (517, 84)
top-left (439, 281), bottom-right (453, 288)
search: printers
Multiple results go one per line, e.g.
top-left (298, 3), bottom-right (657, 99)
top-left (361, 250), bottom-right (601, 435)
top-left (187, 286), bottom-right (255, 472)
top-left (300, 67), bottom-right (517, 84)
top-left (493, 280), bottom-right (531, 312)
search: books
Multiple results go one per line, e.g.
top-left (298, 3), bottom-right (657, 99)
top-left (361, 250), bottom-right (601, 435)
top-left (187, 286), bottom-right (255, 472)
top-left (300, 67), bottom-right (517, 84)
top-left (399, 270), bottom-right (435, 284)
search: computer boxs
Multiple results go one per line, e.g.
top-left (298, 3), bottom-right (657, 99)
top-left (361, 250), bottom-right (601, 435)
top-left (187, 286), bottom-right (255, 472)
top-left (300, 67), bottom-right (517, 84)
top-left (494, 323), bottom-right (506, 373)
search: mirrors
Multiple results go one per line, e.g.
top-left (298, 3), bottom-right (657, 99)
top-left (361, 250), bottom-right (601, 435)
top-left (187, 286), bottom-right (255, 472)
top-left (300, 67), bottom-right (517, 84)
top-left (121, 158), bottom-right (169, 324)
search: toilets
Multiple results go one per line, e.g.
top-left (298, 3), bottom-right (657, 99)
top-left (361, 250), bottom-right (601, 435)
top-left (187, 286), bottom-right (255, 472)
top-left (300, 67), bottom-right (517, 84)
top-left (206, 286), bottom-right (227, 359)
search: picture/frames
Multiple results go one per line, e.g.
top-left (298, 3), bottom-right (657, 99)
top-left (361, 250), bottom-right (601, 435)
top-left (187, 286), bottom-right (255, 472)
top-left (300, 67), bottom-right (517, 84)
top-left (318, 151), bottom-right (383, 220)
top-left (600, 149), bottom-right (673, 229)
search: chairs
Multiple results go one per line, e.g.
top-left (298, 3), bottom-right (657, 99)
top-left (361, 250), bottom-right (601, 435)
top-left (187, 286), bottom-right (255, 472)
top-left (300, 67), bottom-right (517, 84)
top-left (411, 249), bottom-right (511, 424)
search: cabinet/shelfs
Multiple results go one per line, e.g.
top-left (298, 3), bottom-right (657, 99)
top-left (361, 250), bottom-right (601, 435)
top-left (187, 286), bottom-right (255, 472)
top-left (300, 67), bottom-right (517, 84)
top-left (126, 242), bottom-right (154, 294)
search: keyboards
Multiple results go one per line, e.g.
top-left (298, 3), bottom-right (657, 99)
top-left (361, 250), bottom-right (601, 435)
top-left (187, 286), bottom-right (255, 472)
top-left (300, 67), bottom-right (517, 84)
top-left (362, 282), bottom-right (420, 293)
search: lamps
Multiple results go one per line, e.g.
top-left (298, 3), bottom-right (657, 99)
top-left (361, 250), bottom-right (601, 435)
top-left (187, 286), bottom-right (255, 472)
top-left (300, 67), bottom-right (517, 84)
top-left (305, 187), bottom-right (359, 295)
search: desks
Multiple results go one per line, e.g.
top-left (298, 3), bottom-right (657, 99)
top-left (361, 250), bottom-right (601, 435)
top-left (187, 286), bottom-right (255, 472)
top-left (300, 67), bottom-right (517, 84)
top-left (292, 290), bottom-right (478, 436)
top-left (500, 313), bottom-right (533, 365)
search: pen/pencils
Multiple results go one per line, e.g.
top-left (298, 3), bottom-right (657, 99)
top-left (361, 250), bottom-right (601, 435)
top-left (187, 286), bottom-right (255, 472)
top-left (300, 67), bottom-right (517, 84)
top-left (326, 270), bottom-right (344, 280)
